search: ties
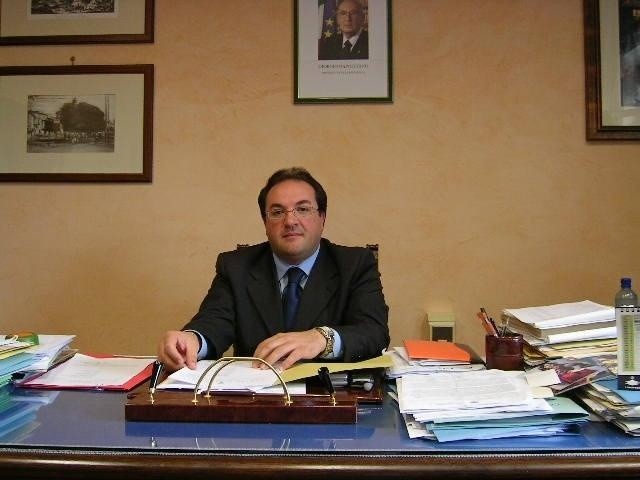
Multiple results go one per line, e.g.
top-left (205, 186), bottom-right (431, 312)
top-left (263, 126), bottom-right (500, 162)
top-left (343, 40), bottom-right (352, 55)
top-left (281, 267), bottom-right (305, 332)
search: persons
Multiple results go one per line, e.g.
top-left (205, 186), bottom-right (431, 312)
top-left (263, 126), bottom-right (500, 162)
top-left (317, 0), bottom-right (369, 59)
top-left (160, 167), bottom-right (390, 373)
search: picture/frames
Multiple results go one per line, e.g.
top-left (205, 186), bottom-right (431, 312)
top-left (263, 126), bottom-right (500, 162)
top-left (0, 0), bottom-right (154, 184)
top-left (582, 1), bottom-right (640, 142)
top-left (292, 0), bottom-right (393, 105)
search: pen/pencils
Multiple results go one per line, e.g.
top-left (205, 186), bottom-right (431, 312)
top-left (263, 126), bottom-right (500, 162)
top-left (477, 307), bottom-right (515, 337)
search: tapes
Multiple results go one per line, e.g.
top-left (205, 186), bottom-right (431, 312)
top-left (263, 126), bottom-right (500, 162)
top-left (5, 333), bottom-right (39, 345)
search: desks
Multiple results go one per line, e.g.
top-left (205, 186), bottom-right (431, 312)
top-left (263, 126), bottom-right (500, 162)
top-left (1, 354), bottom-right (640, 479)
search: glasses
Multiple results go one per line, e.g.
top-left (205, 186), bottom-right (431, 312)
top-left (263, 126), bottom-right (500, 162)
top-left (264, 205), bottom-right (319, 220)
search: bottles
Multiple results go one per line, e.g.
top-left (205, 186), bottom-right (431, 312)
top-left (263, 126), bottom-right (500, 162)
top-left (615, 277), bottom-right (637, 322)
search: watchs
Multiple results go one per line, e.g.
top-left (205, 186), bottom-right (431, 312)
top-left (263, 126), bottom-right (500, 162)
top-left (314, 323), bottom-right (335, 360)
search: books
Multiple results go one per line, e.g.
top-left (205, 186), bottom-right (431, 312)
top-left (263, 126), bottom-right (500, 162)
top-left (0, 327), bottom-right (162, 392)
top-left (388, 297), bottom-right (640, 442)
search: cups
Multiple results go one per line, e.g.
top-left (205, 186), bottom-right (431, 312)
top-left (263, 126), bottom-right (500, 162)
top-left (484, 332), bottom-right (524, 371)
